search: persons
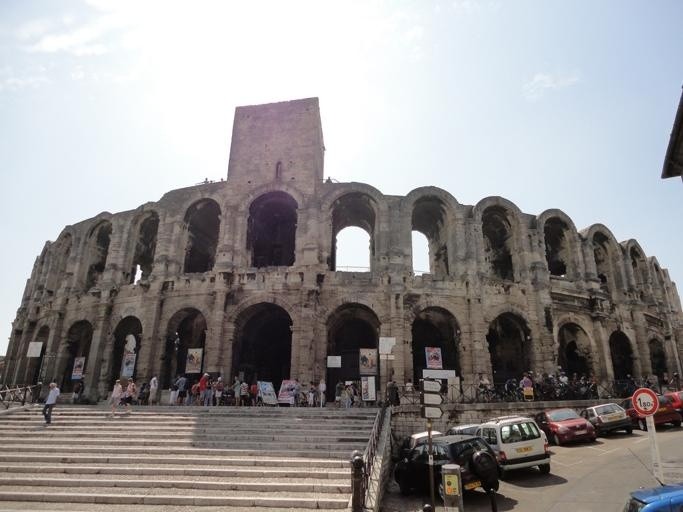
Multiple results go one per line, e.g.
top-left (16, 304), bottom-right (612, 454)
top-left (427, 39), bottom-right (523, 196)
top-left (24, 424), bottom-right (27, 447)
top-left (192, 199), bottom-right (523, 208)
top-left (477, 373), bottom-right (491, 403)
top-left (138, 371), bottom-right (362, 408)
top-left (325, 176), bottom-right (332, 183)
top-left (72, 375), bottom-right (86, 405)
top-left (123, 378), bottom-right (138, 412)
top-left (109, 380), bottom-right (123, 416)
top-left (388, 380), bottom-right (401, 406)
top-left (502, 368), bottom-right (597, 402)
top-left (41, 381), bottom-right (61, 427)
top-left (404, 378), bottom-right (416, 391)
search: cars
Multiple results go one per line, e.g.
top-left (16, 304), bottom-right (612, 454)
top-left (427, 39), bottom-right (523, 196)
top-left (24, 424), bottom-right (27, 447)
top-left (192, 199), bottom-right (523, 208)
top-left (405, 430), bottom-right (444, 449)
top-left (534, 407), bottom-right (597, 445)
top-left (578, 402), bottom-right (632, 436)
top-left (446, 423), bottom-right (479, 436)
top-left (619, 393), bottom-right (682, 431)
top-left (623, 482), bottom-right (683, 511)
top-left (665, 391), bottom-right (682, 414)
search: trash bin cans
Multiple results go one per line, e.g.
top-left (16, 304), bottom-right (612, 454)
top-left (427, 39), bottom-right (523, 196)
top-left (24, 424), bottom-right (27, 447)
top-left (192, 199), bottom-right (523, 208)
top-left (442, 464), bottom-right (464, 512)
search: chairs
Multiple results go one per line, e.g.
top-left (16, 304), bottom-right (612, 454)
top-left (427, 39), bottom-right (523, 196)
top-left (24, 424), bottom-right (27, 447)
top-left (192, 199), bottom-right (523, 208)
top-left (506, 430), bottom-right (522, 442)
top-left (476, 381), bottom-right (601, 401)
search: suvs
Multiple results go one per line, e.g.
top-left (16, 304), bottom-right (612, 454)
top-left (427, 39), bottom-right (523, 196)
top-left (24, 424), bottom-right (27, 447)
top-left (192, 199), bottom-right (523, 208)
top-left (394, 434), bottom-right (500, 502)
top-left (471, 415), bottom-right (551, 480)
